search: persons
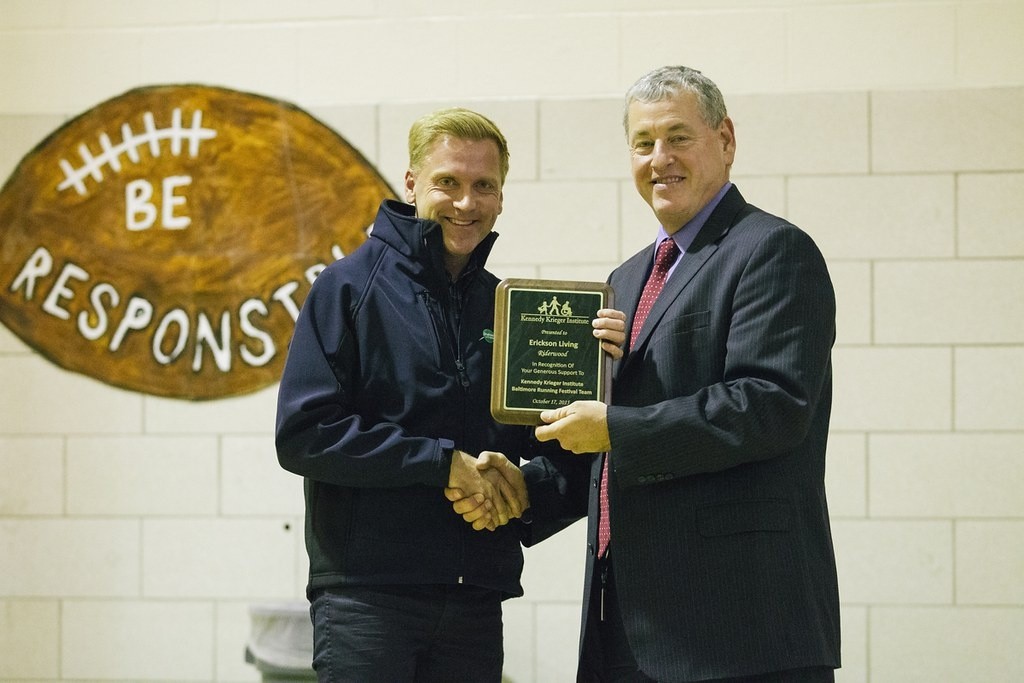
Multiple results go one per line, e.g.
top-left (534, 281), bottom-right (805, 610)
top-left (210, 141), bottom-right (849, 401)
top-left (274, 107), bottom-right (626, 683)
top-left (443, 65), bottom-right (842, 683)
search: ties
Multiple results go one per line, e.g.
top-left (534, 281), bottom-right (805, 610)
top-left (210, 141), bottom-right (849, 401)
top-left (597, 241), bottom-right (682, 559)
